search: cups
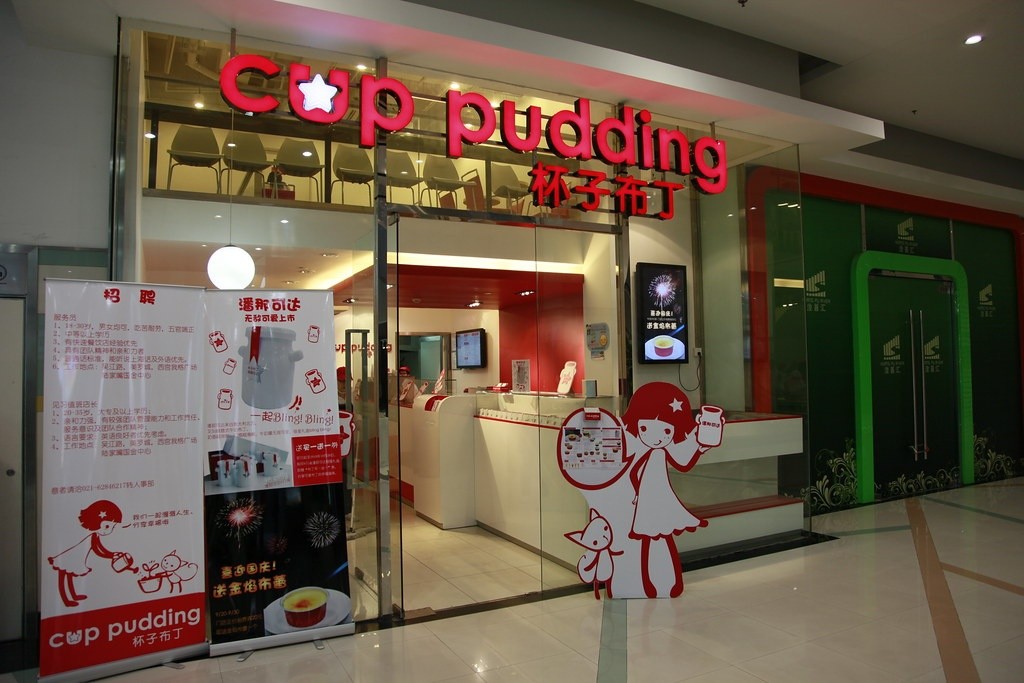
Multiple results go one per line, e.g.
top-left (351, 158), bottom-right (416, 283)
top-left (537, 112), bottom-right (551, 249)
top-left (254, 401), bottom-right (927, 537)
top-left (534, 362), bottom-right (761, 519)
top-left (653, 336), bottom-right (674, 356)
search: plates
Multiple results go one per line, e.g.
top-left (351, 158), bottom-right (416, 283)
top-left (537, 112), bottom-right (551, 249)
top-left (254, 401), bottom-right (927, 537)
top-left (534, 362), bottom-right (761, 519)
top-left (263, 587), bottom-right (351, 635)
top-left (645, 338), bottom-right (685, 359)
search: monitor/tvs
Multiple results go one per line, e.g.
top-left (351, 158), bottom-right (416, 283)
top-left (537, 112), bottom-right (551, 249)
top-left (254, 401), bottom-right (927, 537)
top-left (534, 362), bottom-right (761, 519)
top-left (456, 328), bottom-right (487, 369)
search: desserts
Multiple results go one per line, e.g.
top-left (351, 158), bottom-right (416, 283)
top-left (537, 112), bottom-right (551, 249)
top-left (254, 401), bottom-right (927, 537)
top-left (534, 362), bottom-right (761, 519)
top-left (279, 587), bottom-right (330, 627)
top-left (654, 340), bottom-right (673, 357)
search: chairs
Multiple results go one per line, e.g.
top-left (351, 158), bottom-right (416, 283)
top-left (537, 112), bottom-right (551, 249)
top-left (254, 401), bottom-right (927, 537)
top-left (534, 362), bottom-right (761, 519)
top-left (420, 154), bottom-right (478, 212)
top-left (490, 162), bottom-right (531, 215)
top-left (218, 130), bottom-right (274, 197)
top-left (273, 138), bottom-right (327, 203)
top-left (437, 189), bottom-right (458, 209)
top-left (461, 168), bottom-right (500, 212)
top-left (330, 144), bottom-right (376, 207)
top-left (166, 124), bottom-right (224, 194)
top-left (385, 149), bottom-right (425, 206)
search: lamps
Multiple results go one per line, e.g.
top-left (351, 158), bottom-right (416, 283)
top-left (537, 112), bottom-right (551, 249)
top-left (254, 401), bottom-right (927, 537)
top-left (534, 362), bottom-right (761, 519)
top-left (207, 109), bottom-right (255, 290)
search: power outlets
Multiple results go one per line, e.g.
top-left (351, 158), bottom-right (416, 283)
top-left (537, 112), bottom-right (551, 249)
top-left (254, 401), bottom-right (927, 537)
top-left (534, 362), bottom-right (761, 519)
top-left (693, 348), bottom-right (702, 356)
